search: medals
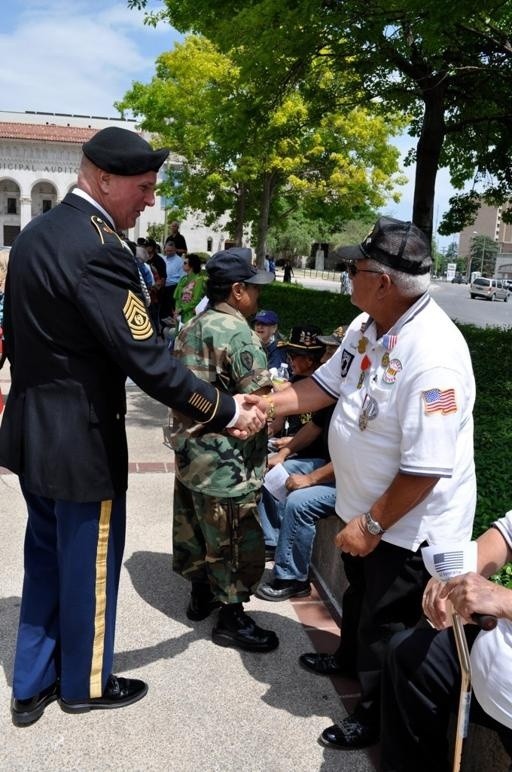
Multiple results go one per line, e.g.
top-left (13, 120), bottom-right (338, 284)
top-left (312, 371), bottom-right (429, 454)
top-left (357, 394), bottom-right (378, 432)
top-left (356, 356), bottom-right (369, 390)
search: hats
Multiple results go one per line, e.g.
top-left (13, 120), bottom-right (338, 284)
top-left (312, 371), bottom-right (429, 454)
top-left (81, 127), bottom-right (170, 177)
top-left (334, 217), bottom-right (435, 276)
top-left (252, 310), bottom-right (279, 325)
top-left (276, 327), bottom-right (325, 349)
top-left (317, 326), bottom-right (348, 346)
top-left (205, 247), bottom-right (275, 285)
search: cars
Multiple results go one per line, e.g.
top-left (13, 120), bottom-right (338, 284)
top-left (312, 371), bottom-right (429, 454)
top-left (451, 276), bottom-right (468, 285)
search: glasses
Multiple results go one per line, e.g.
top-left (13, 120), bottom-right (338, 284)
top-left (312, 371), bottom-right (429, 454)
top-left (183, 262), bottom-right (189, 265)
top-left (286, 353), bottom-right (309, 362)
top-left (348, 262), bottom-right (384, 278)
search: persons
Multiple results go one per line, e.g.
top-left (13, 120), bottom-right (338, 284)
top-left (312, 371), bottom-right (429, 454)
top-left (282, 259), bottom-right (294, 283)
top-left (162, 254), bottom-right (205, 340)
top-left (166, 220), bottom-right (187, 253)
top-left (401, 505), bottom-right (510, 772)
top-left (261, 253), bottom-right (276, 283)
top-left (249, 307), bottom-right (290, 375)
top-left (334, 260), bottom-right (354, 298)
top-left (253, 325), bottom-right (352, 602)
top-left (168, 246), bottom-right (296, 656)
top-left (230, 215), bottom-right (477, 766)
top-left (262, 324), bottom-right (327, 464)
top-left (127, 230), bottom-right (165, 322)
top-left (4, 126), bottom-right (264, 731)
top-left (158, 240), bottom-right (187, 310)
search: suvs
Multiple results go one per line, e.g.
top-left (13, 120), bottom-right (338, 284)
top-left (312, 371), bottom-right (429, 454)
top-left (469, 277), bottom-right (511, 302)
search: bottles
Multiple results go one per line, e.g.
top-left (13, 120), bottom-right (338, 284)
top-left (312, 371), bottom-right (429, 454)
top-left (271, 362), bottom-right (291, 390)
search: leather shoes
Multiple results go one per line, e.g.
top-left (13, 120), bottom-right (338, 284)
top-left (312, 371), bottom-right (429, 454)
top-left (299, 652), bottom-right (358, 677)
top-left (254, 579), bottom-right (311, 601)
top-left (60, 674), bottom-right (149, 714)
top-left (320, 704), bottom-right (398, 751)
top-left (186, 591), bottom-right (226, 623)
top-left (12, 677), bottom-right (60, 726)
top-left (212, 606), bottom-right (279, 654)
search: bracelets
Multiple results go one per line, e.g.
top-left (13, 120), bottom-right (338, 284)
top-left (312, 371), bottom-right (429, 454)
top-left (254, 392), bottom-right (278, 421)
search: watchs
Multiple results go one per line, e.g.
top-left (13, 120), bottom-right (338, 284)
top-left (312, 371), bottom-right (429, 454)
top-left (357, 507), bottom-right (388, 546)
